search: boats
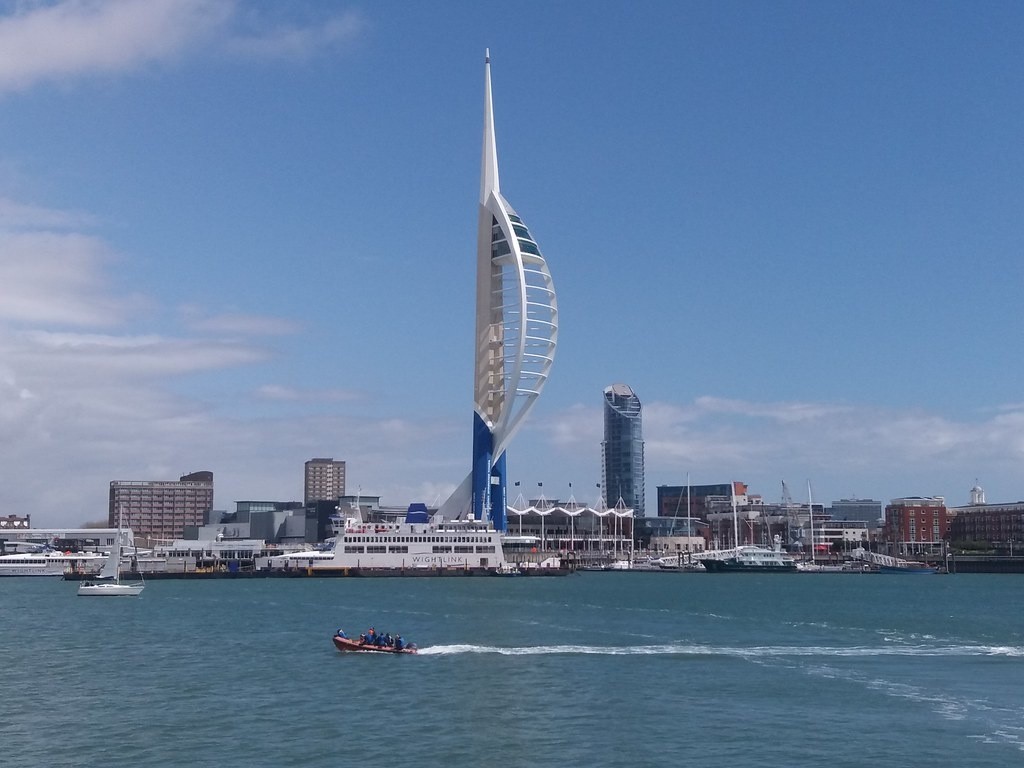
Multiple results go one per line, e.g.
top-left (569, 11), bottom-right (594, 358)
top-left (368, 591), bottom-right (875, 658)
top-left (514, 556), bottom-right (575, 577)
top-left (0, 551), bottom-right (98, 581)
top-left (61, 568), bottom-right (116, 582)
top-left (256, 502), bottom-right (510, 575)
top-left (797, 562), bottom-right (851, 574)
top-left (876, 560), bottom-right (943, 576)
top-left (600, 559), bottom-right (636, 570)
top-left (700, 480), bottom-right (799, 573)
top-left (333, 637), bottom-right (419, 657)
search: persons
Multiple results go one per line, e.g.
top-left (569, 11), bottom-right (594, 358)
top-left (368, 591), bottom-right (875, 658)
top-left (337, 626), bottom-right (405, 650)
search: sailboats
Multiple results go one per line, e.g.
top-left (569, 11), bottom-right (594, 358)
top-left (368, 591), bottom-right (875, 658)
top-left (78, 503), bottom-right (145, 596)
